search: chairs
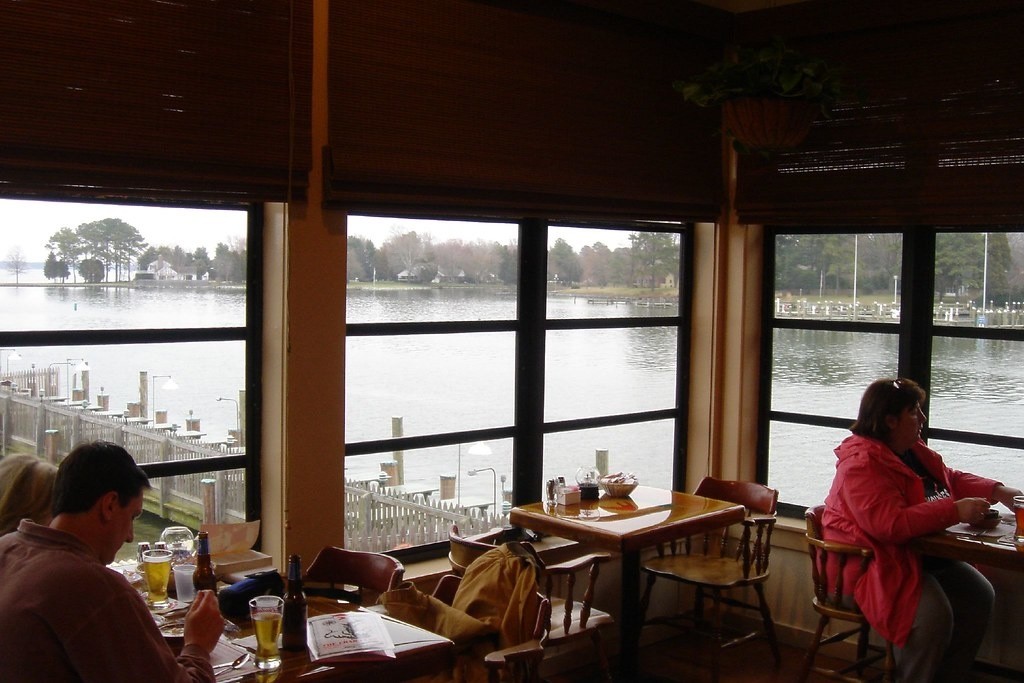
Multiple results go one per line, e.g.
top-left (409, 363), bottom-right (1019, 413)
top-left (797, 505), bottom-right (898, 683)
top-left (297, 475), bottom-right (782, 683)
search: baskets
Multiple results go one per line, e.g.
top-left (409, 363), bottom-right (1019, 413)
top-left (601, 475), bottom-right (639, 497)
top-left (136, 563), bottom-right (196, 591)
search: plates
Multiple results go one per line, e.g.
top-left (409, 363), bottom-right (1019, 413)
top-left (151, 609), bottom-right (187, 639)
top-left (122, 574), bottom-right (141, 584)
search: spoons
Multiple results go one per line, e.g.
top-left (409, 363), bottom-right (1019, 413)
top-left (214, 652), bottom-right (251, 676)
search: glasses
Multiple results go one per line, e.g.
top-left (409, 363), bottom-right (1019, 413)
top-left (888, 379), bottom-right (904, 398)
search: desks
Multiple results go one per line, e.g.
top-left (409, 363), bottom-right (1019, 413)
top-left (911, 515), bottom-right (1024, 572)
top-left (510, 485), bottom-right (747, 683)
top-left (161, 594), bottom-right (456, 683)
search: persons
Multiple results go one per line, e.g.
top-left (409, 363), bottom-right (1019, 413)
top-left (821, 378), bottom-right (1024, 683)
top-left (785, 288), bottom-right (793, 304)
top-left (0, 440), bottom-right (225, 683)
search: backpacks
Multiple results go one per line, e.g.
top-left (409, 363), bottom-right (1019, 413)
top-left (218, 569), bottom-right (285, 619)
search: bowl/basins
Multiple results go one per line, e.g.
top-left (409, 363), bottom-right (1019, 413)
top-left (969, 515), bottom-right (1005, 530)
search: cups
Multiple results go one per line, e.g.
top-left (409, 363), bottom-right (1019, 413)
top-left (249, 595), bottom-right (285, 670)
top-left (1013, 495), bottom-right (1024, 538)
top-left (142, 548), bottom-right (173, 608)
top-left (172, 563), bottom-right (197, 603)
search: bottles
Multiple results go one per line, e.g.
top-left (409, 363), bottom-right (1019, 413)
top-left (136, 525), bottom-right (194, 563)
top-left (193, 532), bottom-right (218, 596)
top-left (545, 477), bottom-right (565, 507)
top-left (281, 554), bottom-right (308, 651)
top-left (575, 465), bottom-right (600, 486)
